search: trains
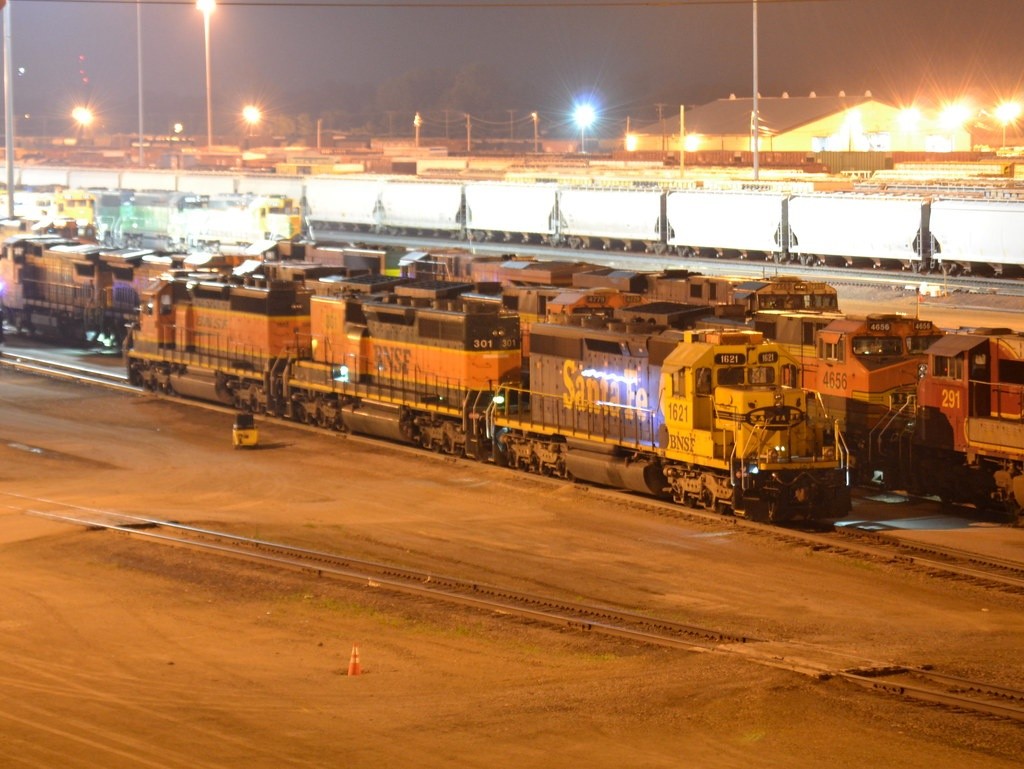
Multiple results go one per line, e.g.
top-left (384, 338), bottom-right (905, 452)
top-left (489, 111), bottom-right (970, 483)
top-left (127, 266), bottom-right (853, 533)
top-left (0, 187), bottom-right (1024, 524)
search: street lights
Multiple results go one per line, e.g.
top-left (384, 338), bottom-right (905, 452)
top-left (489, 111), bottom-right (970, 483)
top-left (572, 101), bottom-right (595, 154)
top-left (531, 113), bottom-right (538, 152)
top-left (195, 1), bottom-right (217, 153)
top-left (414, 113), bottom-right (421, 149)
